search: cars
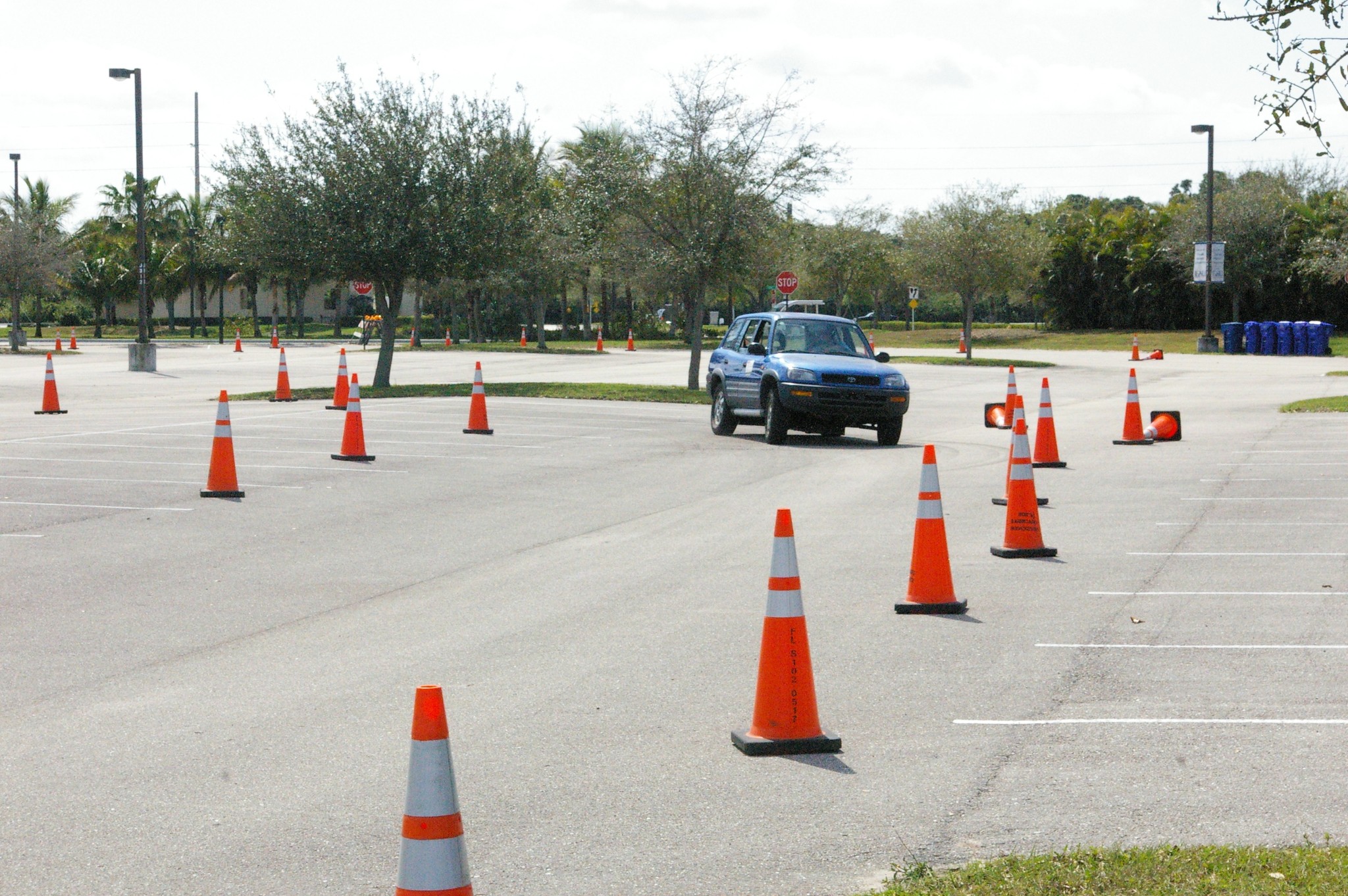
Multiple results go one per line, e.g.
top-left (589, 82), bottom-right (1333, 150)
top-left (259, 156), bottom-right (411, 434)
top-left (851, 311), bottom-right (902, 321)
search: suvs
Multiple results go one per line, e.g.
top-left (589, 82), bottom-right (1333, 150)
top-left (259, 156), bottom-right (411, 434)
top-left (707, 311), bottom-right (910, 447)
top-left (657, 308), bottom-right (666, 320)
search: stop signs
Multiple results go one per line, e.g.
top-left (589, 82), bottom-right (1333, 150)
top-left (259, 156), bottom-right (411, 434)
top-left (353, 276), bottom-right (374, 295)
top-left (777, 270), bottom-right (799, 294)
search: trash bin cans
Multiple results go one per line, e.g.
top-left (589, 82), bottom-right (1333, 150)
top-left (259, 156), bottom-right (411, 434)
top-left (1243, 321), bottom-right (1262, 353)
top-left (1292, 321), bottom-right (1308, 355)
top-left (1276, 321), bottom-right (1294, 354)
top-left (1308, 319), bottom-right (1333, 356)
top-left (1221, 322), bottom-right (1244, 354)
top-left (1260, 321), bottom-right (1278, 355)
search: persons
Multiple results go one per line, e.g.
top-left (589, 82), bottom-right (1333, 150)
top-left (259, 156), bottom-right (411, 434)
top-left (811, 326), bottom-right (852, 354)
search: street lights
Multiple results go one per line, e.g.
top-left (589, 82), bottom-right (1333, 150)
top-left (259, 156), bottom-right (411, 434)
top-left (1192, 125), bottom-right (1220, 352)
top-left (9, 153), bottom-right (28, 347)
top-left (109, 68), bottom-right (158, 372)
top-left (234, 330), bottom-right (244, 352)
top-left (33, 352), bottom-right (68, 413)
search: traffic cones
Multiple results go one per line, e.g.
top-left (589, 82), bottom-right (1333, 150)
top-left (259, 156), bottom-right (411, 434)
top-left (868, 332), bottom-right (875, 356)
top-left (330, 373), bottom-right (375, 461)
top-left (70, 325), bottom-right (78, 349)
top-left (463, 362), bottom-right (494, 435)
top-left (200, 389), bottom-right (246, 498)
top-left (325, 348), bottom-right (351, 410)
top-left (270, 323), bottom-right (280, 349)
top-left (893, 445), bottom-right (968, 614)
top-left (984, 365), bottom-right (1067, 558)
top-left (519, 328), bottom-right (527, 347)
top-left (626, 329), bottom-right (636, 351)
top-left (595, 327), bottom-right (608, 354)
top-left (444, 327), bottom-right (452, 347)
top-left (956, 327), bottom-right (968, 353)
top-left (1128, 332), bottom-right (1163, 361)
top-left (268, 347), bottom-right (298, 402)
top-left (730, 509), bottom-right (842, 756)
top-left (1113, 369), bottom-right (1181, 445)
top-left (395, 684), bottom-right (474, 896)
top-left (54, 328), bottom-right (64, 352)
top-left (409, 327), bottom-right (415, 347)
